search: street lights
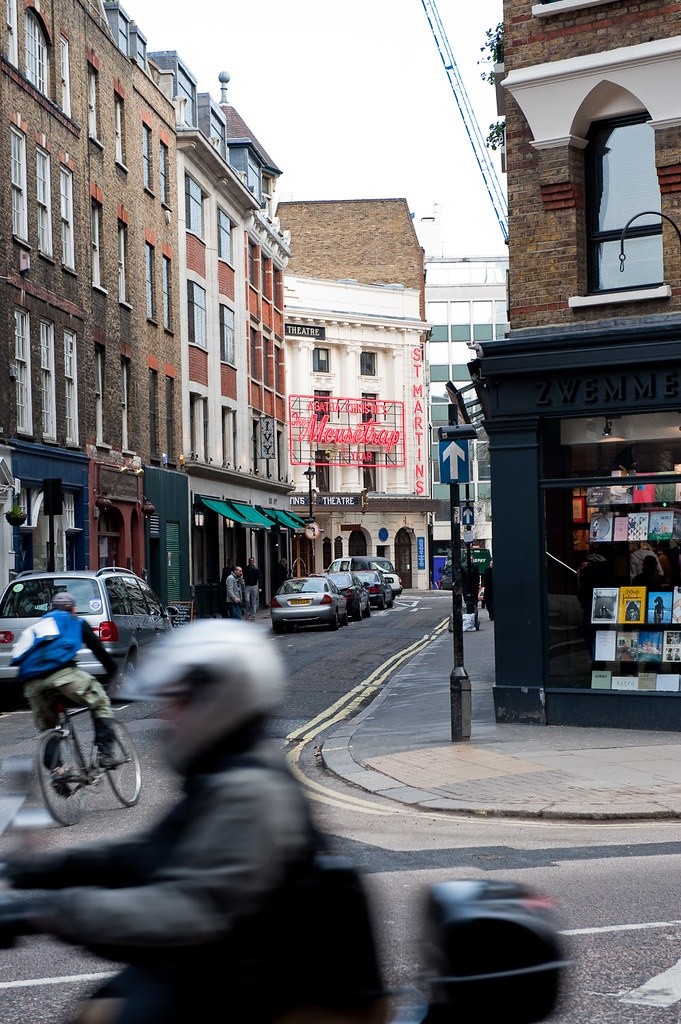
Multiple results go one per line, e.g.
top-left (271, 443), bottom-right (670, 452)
top-left (303, 463), bottom-right (317, 573)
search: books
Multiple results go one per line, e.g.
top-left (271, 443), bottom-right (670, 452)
top-left (591, 587), bottom-right (681, 623)
top-left (595, 631), bottom-right (681, 663)
top-left (587, 465), bottom-right (681, 541)
top-left (592, 671), bottom-right (681, 692)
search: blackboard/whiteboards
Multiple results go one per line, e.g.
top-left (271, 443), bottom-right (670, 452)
top-left (169, 601), bottom-right (194, 630)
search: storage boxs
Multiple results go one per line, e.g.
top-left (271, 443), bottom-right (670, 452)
top-left (413, 877), bottom-right (567, 1024)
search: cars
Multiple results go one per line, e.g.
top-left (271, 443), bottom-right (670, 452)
top-left (438, 565), bottom-right (452, 590)
top-left (270, 570), bottom-right (394, 634)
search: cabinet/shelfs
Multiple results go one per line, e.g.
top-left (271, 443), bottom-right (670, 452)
top-left (585, 501), bottom-right (681, 694)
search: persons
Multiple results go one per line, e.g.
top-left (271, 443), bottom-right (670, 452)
top-left (21, 594), bottom-right (123, 797)
top-left (0, 620), bottom-right (328, 1024)
top-left (462, 556), bottom-right (480, 615)
top-left (276, 558), bottom-right (288, 587)
top-left (220, 557), bottom-right (260, 620)
top-left (482, 559), bottom-right (493, 621)
top-left (630, 540), bottom-right (681, 591)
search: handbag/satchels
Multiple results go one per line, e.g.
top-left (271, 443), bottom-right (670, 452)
top-left (463, 613), bottom-right (476, 632)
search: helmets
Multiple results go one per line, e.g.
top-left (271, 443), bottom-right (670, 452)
top-left (118, 618), bottom-right (284, 766)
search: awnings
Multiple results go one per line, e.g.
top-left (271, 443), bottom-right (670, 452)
top-left (286, 511), bottom-right (324, 532)
top-left (262, 508), bottom-right (306, 532)
top-left (200, 497), bottom-right (263, 528)
top-left (233, 503), bottom-right (287, 533)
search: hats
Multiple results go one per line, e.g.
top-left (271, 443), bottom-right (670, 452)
top-left (53, 594), bottom-right (76, 610)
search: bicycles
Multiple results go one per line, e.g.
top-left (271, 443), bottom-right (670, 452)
top-left (36, 689), bottom-right (143, 827)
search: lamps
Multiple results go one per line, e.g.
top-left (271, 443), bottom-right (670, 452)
top-left (238, 465), bottom-right (242, 471)
top-left (255, 469), bottom-right (259, 475)
top-left (602, 418), bottom-right (613, 437)
top-left (289, 480), bottom-right (294, 485)
top-left (128, 459), bottom-right (145, 479)
top-left (209, 457), bottom-right (213, 464)
top-left (113, 457), bottom-right (129, 476)
top-left (225, 462), bottom-right (230, 469)
top-left (280, 477), bottom-right (283, 481)
top-left (190, 451), bottom-right (195, 461)
top-left (249, 468), bottom-right (252, 475)
top-left (268, 473), bottom-right (272, 478)
top-left (196, 453), bottom-right (198, 461)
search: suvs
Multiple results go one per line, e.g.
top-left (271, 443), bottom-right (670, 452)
top-left (323, 556), bottom-right (404, 600)
top-left (0, 567), bottom-right (179, 703)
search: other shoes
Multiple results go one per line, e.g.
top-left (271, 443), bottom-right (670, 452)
top-left (251, 617), bottom-right (255, 620)
top-left (245, 613), bottom-right (251, 620)
top-left (100, 758), bottom-right (125, 767)
top-left (55, 785), bottom-right (71, 800)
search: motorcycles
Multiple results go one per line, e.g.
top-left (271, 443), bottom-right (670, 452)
top-left (0, 850), bottom-right (567, 1024)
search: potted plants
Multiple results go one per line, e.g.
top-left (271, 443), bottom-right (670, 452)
top-left (485, 118), bottom-right (507, 153)
top-left (477, 21), bottom-right (504, 86)
top-left (4, 499), bottom-right (27, 526)
